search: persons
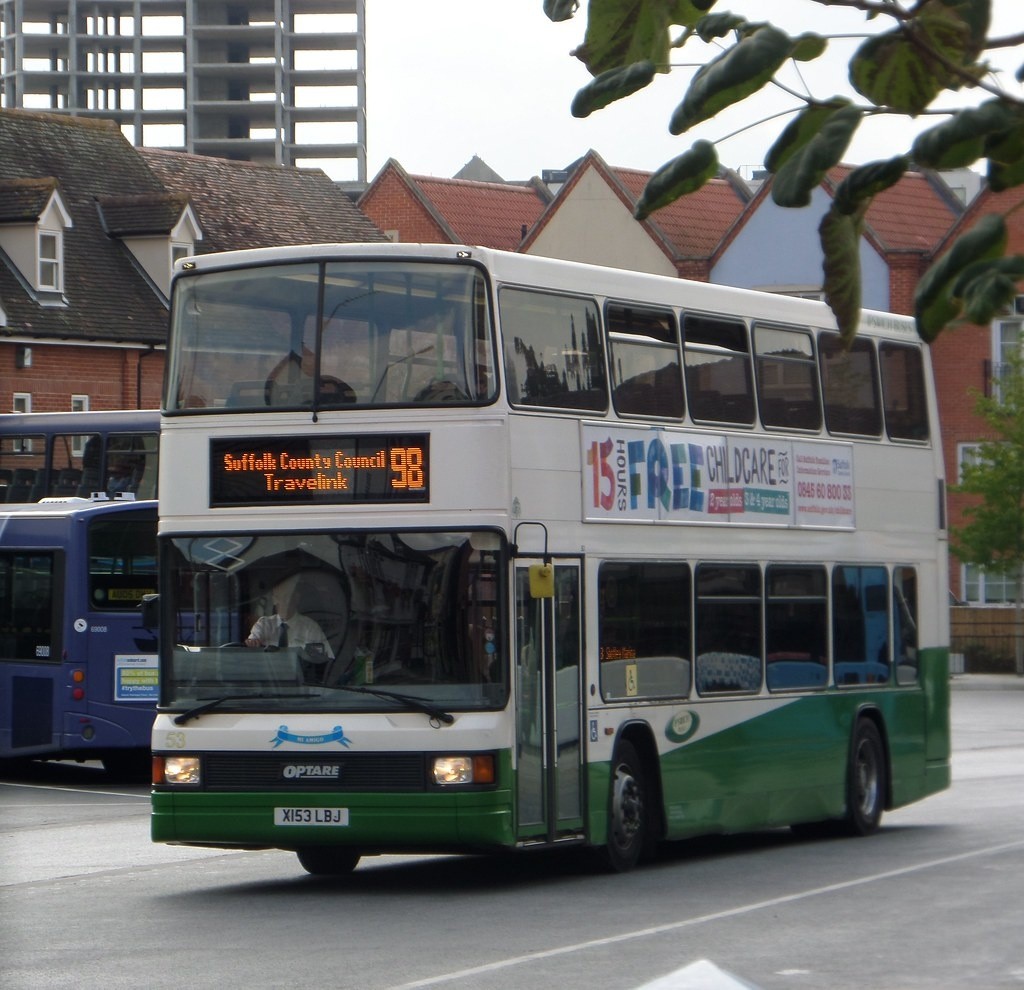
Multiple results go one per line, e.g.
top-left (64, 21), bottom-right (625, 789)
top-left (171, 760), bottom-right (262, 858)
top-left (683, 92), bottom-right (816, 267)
top-left (81, 434), bottom-right (145, 502)
top-left (412, 364), bottom-right (493, 402)
top-left (245, 582), bottom-right (336, 659)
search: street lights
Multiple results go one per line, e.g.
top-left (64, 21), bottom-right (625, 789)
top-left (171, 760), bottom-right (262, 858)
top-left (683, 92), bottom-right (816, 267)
top-left (370, 346), bottom-right (433, 401)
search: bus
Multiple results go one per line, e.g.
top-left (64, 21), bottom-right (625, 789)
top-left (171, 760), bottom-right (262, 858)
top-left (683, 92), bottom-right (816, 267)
top-left (140, 241), bottom-right (949, 876)
top-left (0, 491), bottom-right (247, 782)
top-left (1, 412), bottom-right (163, 573)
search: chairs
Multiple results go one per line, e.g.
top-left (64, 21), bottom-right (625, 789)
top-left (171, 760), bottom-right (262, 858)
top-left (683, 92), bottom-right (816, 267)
top-left (762, 398), bottom-right (787, 426)
top-left (601, 657), bottom-right (690, 697)
top-left (697, 651), bottom-right (761, 690)
top-left (848, 407), bottom-right (874, 434)
top-left (723, 394), bottom-right (753, 422)
top-left (0, 469), bottom-right (81, 503)
top-left (788, 401), bottom-right (819, 429)
top-left (767, 661), bottom-right (827, 687)
top-left (835, 662), bottom-right (889, 685)
top-left (615, 381), bottom-right (659, 414)
top-left (692, 390), bottom-right (721, 420)
top-left (225, 380), bottom-right (277, 407)
